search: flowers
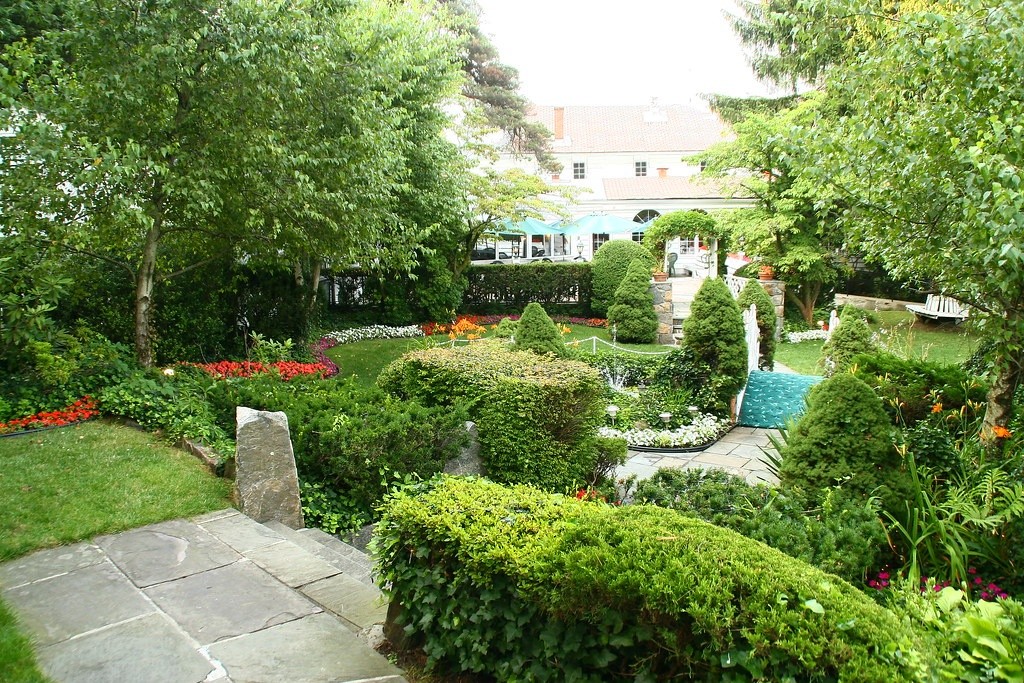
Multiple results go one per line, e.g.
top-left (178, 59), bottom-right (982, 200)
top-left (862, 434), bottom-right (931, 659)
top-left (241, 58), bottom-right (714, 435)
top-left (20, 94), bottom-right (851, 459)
top-left (756, 265), bottom-right (776, 275)
top-left (654, 243), bottom-right (666, 272)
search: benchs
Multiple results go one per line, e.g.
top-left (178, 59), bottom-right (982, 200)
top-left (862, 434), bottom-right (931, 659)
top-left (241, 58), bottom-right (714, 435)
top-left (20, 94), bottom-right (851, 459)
top-left (905, 305), bottom-right (968, 320)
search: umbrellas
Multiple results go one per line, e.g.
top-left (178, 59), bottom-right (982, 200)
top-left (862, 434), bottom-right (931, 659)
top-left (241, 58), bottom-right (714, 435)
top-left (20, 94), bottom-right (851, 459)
top-left (483, 217), bottom-right (564, 259)
top-left (634, 217), bottom-right (660, 233)
top-left (560, 212), bottom-right (642, 245)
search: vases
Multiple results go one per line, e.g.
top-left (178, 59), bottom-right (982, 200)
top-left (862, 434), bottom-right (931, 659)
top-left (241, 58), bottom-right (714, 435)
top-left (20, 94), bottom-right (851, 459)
top-left (759, 274), bottom-right (774, 280)
top-left (653, 274), bottom-right (668, 281)
